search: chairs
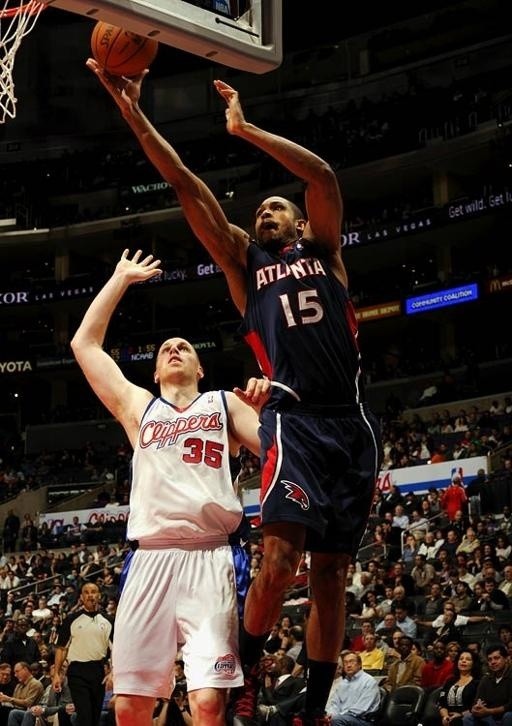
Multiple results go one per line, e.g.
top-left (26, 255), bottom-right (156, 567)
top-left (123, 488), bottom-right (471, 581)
top-left (280, 558), bottom-right (512, 726)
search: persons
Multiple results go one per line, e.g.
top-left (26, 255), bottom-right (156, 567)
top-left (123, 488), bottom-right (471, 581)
top-left (85, 55), bottom-right (384, 725)
top-left (71, 249), bottom-right (274, 726)
top-left (2, 396), bottom-right (511, 726)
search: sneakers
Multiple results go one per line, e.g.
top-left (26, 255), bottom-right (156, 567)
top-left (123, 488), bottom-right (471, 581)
top-left (226, 664), bottom-right (260, 726)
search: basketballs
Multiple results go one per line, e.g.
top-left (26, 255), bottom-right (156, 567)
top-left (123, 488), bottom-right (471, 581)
top-left (92, 22), bottom-right (157, 75)
